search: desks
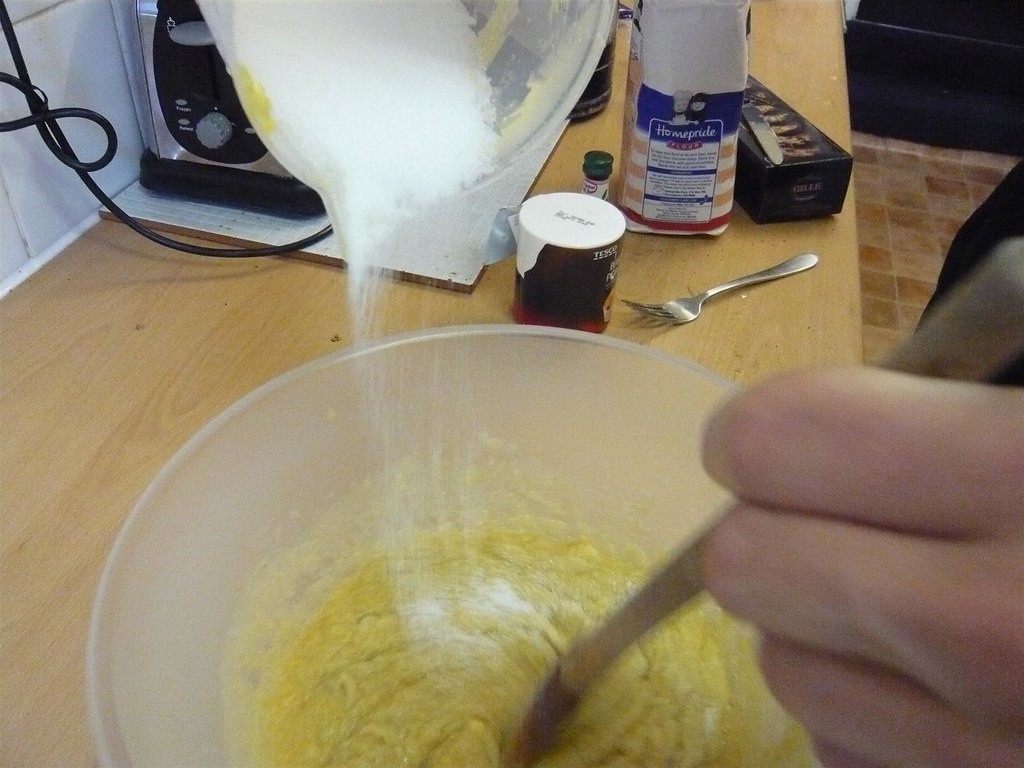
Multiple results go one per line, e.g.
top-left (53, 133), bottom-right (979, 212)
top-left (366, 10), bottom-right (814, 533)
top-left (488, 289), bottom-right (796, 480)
top-left (0, 1), bottom-right (861, 768)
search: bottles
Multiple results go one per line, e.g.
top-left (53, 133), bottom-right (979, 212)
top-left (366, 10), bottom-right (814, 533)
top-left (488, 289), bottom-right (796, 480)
top-left (616, 0), bottom-right (753, 237)
top-left (580, 151), bottom-right (615, 203)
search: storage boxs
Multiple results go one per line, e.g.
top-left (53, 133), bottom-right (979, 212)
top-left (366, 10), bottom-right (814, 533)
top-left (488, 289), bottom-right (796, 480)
top-left (735, 73), bottom-right (853, 224)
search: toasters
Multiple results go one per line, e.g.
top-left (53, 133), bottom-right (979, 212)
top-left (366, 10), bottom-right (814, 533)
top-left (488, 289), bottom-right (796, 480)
top-left (131, 0), bottom-right (324, 219)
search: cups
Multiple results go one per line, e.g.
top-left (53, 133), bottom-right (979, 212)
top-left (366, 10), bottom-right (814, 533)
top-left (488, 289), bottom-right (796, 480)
top-left (194, 1), bottom-right (617, 206)
top-left (510, 193), bottom-right (625, 334)
top-left (82, 322), bottom-right (822, 768)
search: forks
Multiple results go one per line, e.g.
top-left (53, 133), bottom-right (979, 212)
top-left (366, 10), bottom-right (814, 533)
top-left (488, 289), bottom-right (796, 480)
top-left (620, 252), bottom-right (818, 325)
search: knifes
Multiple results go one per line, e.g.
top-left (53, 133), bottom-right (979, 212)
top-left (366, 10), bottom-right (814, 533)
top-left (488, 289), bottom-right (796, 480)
top-left (741, 92), bottom-right (784, 165)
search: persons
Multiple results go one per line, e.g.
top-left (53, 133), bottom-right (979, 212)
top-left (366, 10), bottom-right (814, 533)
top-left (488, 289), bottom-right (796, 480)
top-left (695, 158), bottom-right (1024, 767)
